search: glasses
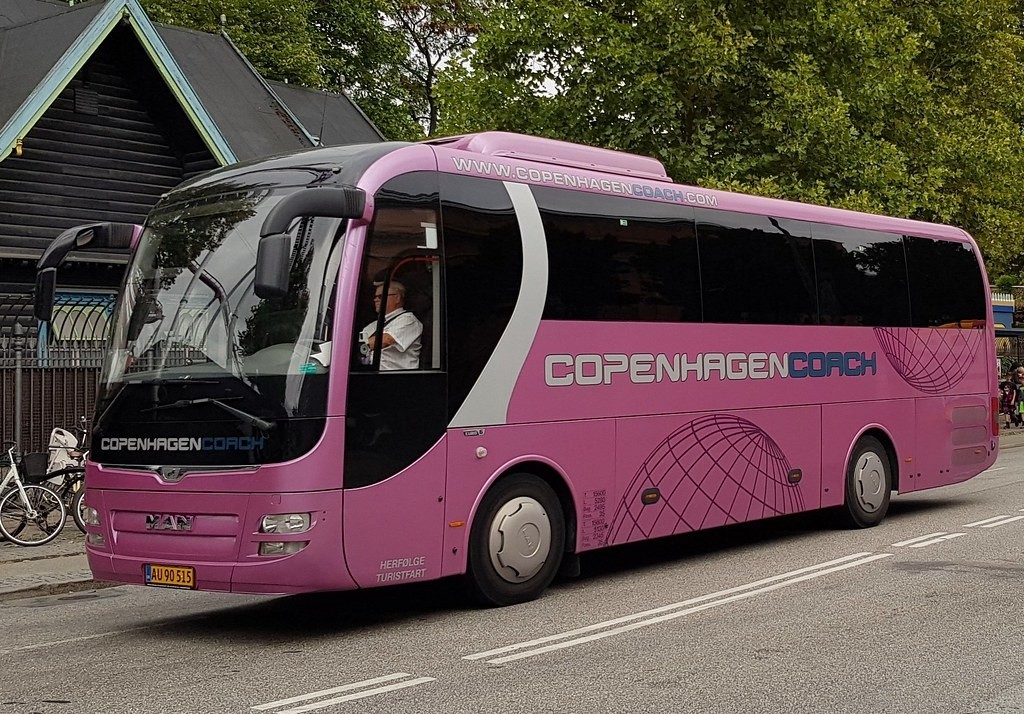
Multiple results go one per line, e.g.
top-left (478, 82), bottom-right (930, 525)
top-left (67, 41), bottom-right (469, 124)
top-left (375, 293), bottom-right (399, 299)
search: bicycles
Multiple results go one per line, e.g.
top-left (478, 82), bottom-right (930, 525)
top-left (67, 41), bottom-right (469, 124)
top-left (0, 440), bottom-right (67, 547)
top-left (0, 414), bottom-right (89, 543)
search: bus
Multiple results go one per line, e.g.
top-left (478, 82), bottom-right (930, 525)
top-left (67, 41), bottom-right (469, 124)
top-left (32, 130), bottom-right (1000, 607)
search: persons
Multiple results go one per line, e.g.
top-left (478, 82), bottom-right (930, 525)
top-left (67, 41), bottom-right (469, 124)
top-left (999, 366), bottom-right (1024, 428)
top-left (358, 281), bottom-right (426, 372)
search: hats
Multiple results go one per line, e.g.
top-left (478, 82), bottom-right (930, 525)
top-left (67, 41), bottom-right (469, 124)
top-left (374, 281), bottom-right (406, 296)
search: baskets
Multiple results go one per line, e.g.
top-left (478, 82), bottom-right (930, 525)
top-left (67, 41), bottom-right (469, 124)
top-left (18, 452), bottom-right (49, 482)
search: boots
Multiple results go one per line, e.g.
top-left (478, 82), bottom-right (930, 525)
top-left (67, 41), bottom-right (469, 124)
top-left (1004, 422), bottom-right (1010, 429)
top-left (1013, 417), bottom-right (1019, 427)
